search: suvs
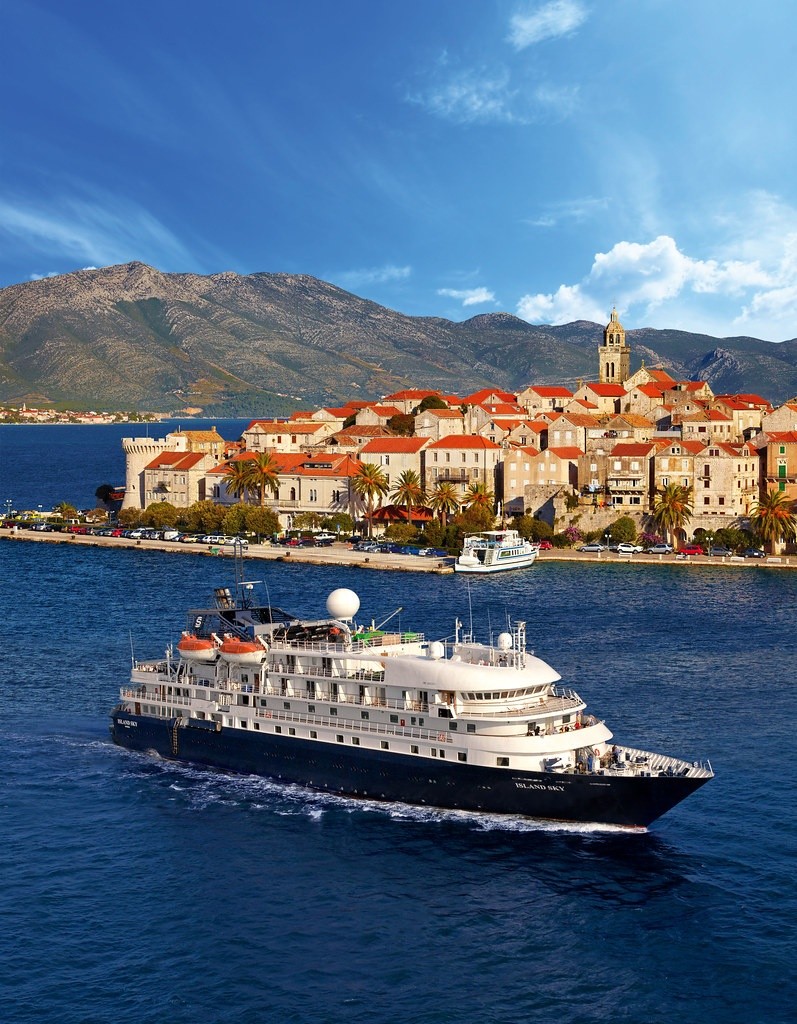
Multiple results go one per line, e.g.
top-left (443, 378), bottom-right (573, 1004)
top-left (644, 543), bottom-right (673, 555)
top-left (616, 543), bottom-right (643, 554)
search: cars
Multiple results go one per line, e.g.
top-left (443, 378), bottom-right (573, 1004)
top-left (273, 539), bottom-right (332, 547)
top-left (353, 541), bottom-right (447, 558)
top-left (532, 541), bottom-right (552, 550)
top-left (710, 547), bottom-right (732, 557)
top-left (675, 546), bottom-right (703, 555)
top-left (739, 547), bottom-right (766, 559)
top-left (579, 543), bottom-right (604, 553)
top-left (0, 520), bottom-right (249, 545)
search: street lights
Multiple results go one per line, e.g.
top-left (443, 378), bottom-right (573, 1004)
top-left (604, 534), bottom-right (613, 553)
top-left (705, 536), bottom-right (713, 557)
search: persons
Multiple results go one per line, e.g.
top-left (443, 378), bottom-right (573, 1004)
top-left (526, 708), bottom-right (652, 776)
top-left (504, 653), bottom-right (508, 663)
top-left (278, 659), bottom-right (284, 672)
top-left (498, 654), bottom-right (502, 664)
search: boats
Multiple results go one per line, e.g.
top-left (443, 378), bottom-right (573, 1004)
top-left (107, 540), bottom-right (714, 827)
top-left (455, 494), bottom-right (537, 572)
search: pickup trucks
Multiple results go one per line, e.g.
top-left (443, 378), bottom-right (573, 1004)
top-left (344, 536), bottom-right (371, 543)
top-left (312, 533), bottom-right (337, 540)
